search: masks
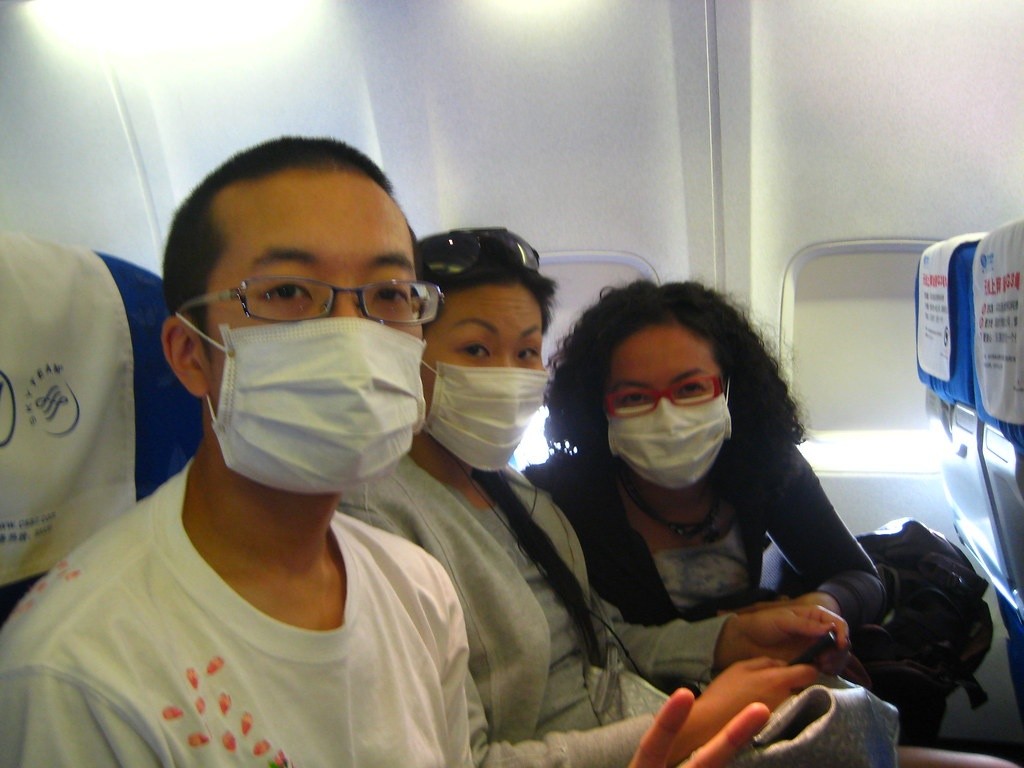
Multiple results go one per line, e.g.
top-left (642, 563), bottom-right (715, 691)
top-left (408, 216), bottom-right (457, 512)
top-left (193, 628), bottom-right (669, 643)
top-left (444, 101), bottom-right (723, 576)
top-left (174, 311), bottom-right (426, 493)
top-left (421, 359), bottom-right (548, 470)
top-left (606, 376), bottom-right (731, 489)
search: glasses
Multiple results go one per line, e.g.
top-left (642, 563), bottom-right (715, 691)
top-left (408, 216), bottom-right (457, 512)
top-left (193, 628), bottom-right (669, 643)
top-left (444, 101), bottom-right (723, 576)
top-left (179, 275), bottom-right (444, 326)
top-left (418, 229), bottom-right (539, 275)
top-left (604, 376), bottom-right (724, 418)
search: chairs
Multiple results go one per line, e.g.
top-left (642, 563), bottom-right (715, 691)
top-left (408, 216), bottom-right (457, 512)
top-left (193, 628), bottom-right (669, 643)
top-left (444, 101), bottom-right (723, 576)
top-left (970, 222), bottom-right (1024, 627)
top-left (913, 232), bottom-right (1024, 716)
top-left (0, 234), bottom-right (214, 639)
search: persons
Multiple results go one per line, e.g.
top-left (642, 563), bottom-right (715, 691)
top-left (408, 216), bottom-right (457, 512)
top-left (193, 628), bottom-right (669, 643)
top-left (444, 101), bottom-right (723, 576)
top-left (520, 277), bottom-right (994, 747)
top-left (0, 137), bottom-right (774, 768)
top-left (334, 224), bottom-right (1015, 768)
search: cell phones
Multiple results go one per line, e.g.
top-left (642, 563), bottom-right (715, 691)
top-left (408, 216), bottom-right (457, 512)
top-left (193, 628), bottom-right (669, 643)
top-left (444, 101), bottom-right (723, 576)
top-left (788, 631), bottom-right (836, 667)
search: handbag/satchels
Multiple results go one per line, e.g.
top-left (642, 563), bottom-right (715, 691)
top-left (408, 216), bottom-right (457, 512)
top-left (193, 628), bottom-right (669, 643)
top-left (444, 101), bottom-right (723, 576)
top-left (583, 588), bottom-right (899, 768)
top-left (855, 520), bottom-right (992, 709)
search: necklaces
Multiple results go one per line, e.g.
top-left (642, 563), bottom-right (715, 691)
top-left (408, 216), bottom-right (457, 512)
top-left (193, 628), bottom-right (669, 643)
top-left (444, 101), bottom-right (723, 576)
top-left (619, 468), bottom-right (726, 542)
